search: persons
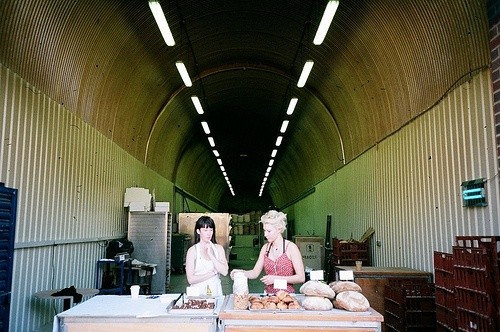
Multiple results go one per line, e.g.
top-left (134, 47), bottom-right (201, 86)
top-left (186, 215), bottom-right (228, 297)
top-left (230, 210), bottom-right (305, 294)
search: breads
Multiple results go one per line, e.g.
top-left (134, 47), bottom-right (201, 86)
top-left (299, 280), bottom-right (369, 313)
top-left (233, 290), bottom-right (300, 310)
top-left (174, 299), bottom-right (215, 309)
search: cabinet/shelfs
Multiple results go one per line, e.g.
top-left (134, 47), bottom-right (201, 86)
top-left (95, 259), bottom-right (154, 295)
top-left (331, 238), bottom-right (370, 267)
top-left (334, 265), bottom-right (433, 316)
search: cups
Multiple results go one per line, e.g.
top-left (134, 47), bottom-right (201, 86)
top-left (130, 285), bottom-right (140, 298)
top-left (356, 261), bottom-right (362, 271)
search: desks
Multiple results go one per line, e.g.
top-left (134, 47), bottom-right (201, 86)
top-left (33, 288), bottom-right (98, 309)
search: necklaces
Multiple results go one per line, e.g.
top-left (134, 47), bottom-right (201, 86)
top-left (275, 246), bottom-right (279, 251)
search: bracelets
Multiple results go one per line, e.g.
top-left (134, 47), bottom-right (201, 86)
top-left (212, 270), bottom-right (216, 275)
top-left (207, 272), bottom-right (212, 279)
